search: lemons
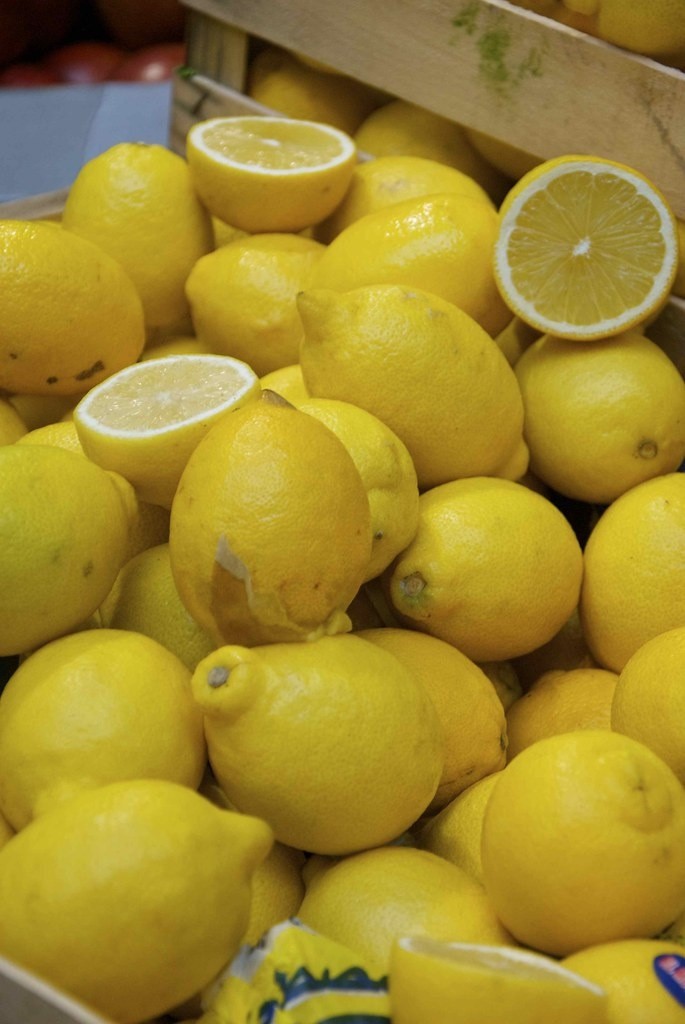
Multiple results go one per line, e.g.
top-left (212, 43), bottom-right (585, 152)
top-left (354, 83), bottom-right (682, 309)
top-left (0, 0), bottom-right (685, 1024)
